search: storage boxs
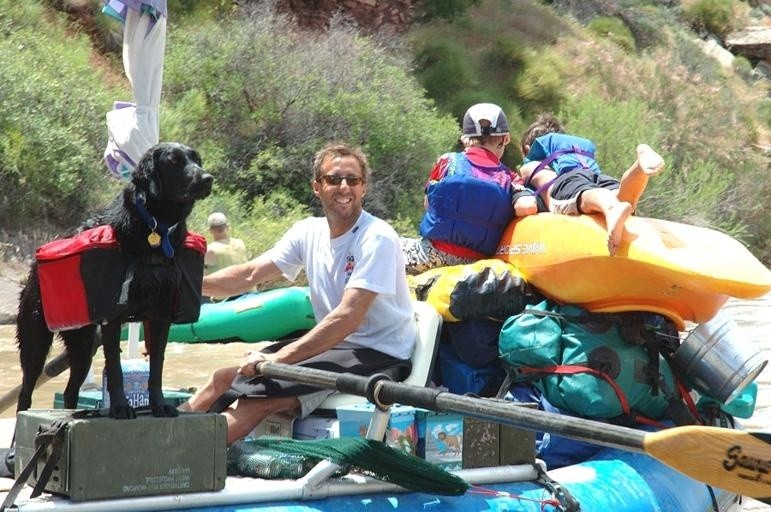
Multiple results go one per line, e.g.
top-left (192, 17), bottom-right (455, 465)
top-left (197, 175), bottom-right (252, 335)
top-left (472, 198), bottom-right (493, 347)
top-left (245, 393), bottom-right (539, 471)
top-left (14, 405), bottom-right (229, 503)
top-left (52, 389), bottom-right (193, 414)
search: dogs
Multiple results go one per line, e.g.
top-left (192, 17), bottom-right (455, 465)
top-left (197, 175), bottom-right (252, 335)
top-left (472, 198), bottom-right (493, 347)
top-left (14, 140), bottom-right (215, 419)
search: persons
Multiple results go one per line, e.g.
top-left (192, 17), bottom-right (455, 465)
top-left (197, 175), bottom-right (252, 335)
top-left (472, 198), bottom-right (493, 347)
top-left (519, 111), bottom-right (666, 258)
top-left (176, 141), bottom-right (419, 446)
top-left (202, 212), bottom-right (246, 303)
top-left (399, 103), bottom-right (538, 276)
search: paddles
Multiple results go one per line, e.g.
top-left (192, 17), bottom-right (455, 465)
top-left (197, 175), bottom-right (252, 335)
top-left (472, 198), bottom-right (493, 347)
top-left (257, 362), bottom-right (759, 501)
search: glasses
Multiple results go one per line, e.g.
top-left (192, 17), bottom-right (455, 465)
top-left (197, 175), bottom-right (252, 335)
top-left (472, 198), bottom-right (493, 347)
top-left (316, 173), bottom-right (366, 188)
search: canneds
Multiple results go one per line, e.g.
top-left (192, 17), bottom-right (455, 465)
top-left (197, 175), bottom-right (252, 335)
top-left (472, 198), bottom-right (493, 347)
top-left (232, 440), bottom-right (305, 480)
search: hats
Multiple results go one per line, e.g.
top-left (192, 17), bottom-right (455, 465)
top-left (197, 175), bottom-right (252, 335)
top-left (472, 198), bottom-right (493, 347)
top-left (207, 212), bottom-right (226, 228)
top-left (460, 100), bottom-right (510, 143)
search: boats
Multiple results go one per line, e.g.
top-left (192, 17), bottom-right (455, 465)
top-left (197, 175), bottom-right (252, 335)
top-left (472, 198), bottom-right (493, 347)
top-left (496, 208), bottom-right (771, 339)
top-left (101, 282), bottom-right (318, 348)
top-left (0, 384), bottom-right (751, 512)
